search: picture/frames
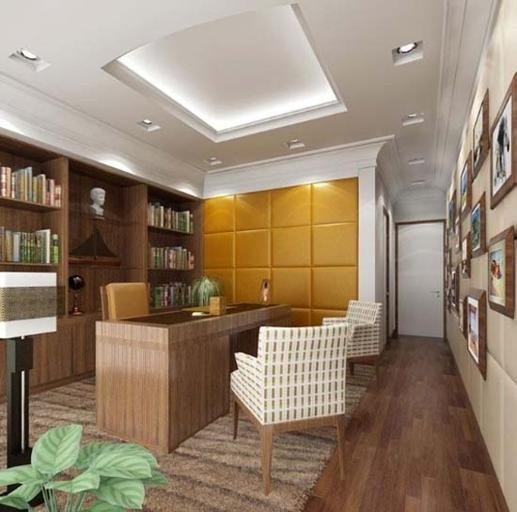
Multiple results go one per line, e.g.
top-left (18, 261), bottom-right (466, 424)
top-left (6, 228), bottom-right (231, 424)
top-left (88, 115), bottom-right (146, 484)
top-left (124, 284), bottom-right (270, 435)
top-left (450, 265), bottom-right (459, 316)
top-left (467, 287), bottom-right (488, 379)
top-left (457, 294), bottom-right (466, 337)
top-left (488, 227), bottom-right (514, 318)
top-left (448, 189), bottom-right (456, 232)
top-left (469, 193), bottom-right (488, 258)
top-left (454, 218), bottom-right (461, 256)
top-left (446, 251), bottom-right (452, 272)
top-left (491, 70), bottom-right (517, 211)
top-left (462, 232), bottom-right (470, 282)
top-left (460, 149), bottom-right (470, 223)
top-left (473, 90), bottom-right (490, 181)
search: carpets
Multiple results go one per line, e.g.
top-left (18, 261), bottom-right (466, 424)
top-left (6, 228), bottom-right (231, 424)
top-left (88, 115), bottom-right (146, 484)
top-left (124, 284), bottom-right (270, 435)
top-left (0, 363), bottom-right (376, 511)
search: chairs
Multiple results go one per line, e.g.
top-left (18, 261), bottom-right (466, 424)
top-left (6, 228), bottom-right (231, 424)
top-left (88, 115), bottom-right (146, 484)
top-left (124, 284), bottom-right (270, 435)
top-left (100, 282), bottom-right (150, 322)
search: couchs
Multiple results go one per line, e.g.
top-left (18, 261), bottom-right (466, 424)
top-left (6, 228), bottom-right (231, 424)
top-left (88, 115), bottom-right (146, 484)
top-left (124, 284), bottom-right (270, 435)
top-left (321, 300), bottom-right (384, 389)
top-left (229, 324), bottom-right (350, 496)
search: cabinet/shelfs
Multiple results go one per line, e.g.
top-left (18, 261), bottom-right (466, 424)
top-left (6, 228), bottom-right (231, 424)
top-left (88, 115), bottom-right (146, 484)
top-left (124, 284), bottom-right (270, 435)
top-left (0, 127), bottom-right (65, 321)
top-left (123, 180), bottom-right (201, 308)
top-left (73, 316), bottom-right (100, 381)
top-left (0, 320), bottom-right (73, 396)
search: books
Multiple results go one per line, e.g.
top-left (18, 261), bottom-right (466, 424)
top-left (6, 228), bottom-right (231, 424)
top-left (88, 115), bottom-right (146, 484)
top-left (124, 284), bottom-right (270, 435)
top-left (150, 245), bottom-right (195, 271)
top-left (1, 226), bottom-right (59, 265)
top-left (147, 202), bottom-right (195, 235)
top-left (0, 166), bottom-right (63, 209)
top-left (154, 280), bottom-right (198, 307)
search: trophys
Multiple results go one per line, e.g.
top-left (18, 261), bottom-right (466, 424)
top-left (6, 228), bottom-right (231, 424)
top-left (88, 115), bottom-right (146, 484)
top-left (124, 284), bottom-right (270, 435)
top-left (67, 274), bottom-right (86, 317)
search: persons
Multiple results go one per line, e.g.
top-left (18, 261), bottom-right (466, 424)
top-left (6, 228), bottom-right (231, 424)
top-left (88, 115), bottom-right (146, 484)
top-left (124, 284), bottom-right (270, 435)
top-left (262, 282), bottom-right (270, 303)
top-left (88, 187), bottom-right (107, 216)
top-left (492, 258), bottom-right (504, 298)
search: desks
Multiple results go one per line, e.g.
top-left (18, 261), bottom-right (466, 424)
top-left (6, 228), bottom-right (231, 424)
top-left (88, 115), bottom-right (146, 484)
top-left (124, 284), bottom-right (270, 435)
top-left (95, 303), bottom-right (289, 454)
